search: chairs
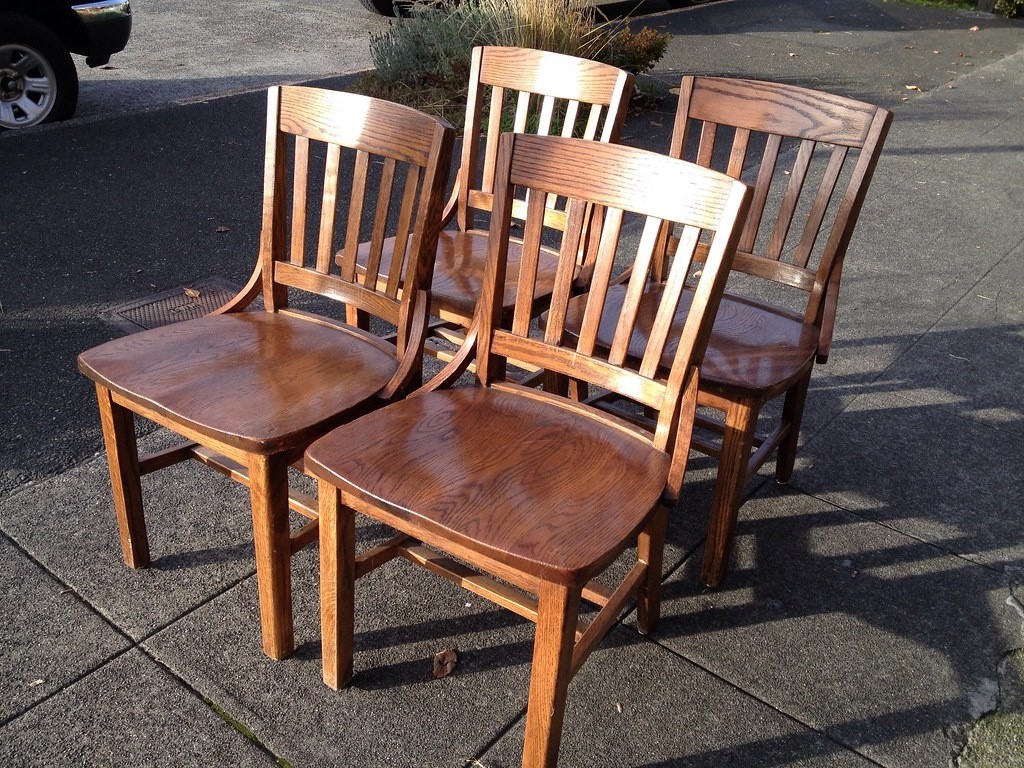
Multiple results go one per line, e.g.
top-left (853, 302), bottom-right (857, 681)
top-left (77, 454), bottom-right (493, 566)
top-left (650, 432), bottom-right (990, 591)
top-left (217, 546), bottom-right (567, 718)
top-left (76, 43), bottom-right (893, 768)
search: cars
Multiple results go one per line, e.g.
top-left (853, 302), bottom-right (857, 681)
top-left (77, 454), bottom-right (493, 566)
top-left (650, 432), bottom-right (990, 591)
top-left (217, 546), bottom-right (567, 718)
top-left (0, 0), bottom-right (134, 133)
